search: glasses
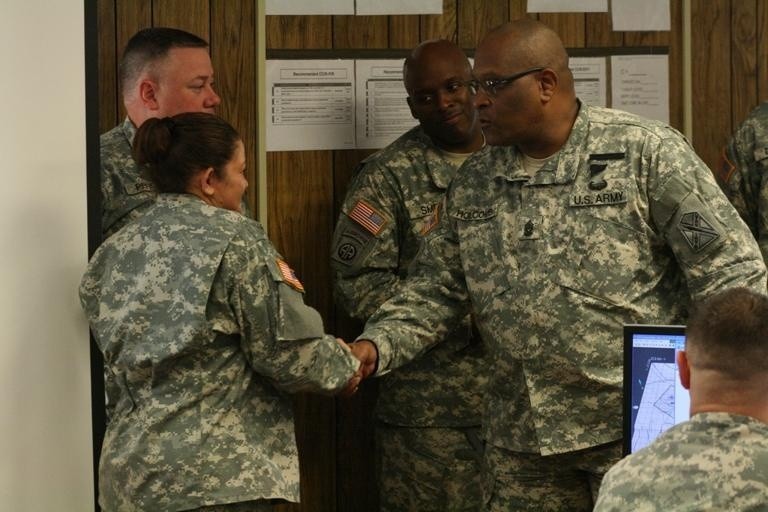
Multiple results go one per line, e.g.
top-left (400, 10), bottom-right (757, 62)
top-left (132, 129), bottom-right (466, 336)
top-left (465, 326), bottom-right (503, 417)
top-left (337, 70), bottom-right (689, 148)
top-left (467, 68), bottom-right (543, 96)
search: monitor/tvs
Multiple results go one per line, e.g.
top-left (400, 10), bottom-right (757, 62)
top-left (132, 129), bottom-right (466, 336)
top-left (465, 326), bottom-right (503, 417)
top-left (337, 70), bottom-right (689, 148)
top-left (622, 323), bottom-right (692, 459)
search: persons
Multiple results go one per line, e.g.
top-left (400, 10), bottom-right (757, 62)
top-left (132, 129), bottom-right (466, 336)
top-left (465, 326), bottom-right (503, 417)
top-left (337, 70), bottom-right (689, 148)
top-left (592, 285), bottom-right (768, 512)
top-left (98, 26), bottom-right (253, 246)
top-left (76, 112), bottom-right (363, 512)
top-left (342, 18), bottom-right (768, 512)
top-left (719, 98), bottom-right (768, 270)
top-left (329, 37), bottom-right (488, 512)
